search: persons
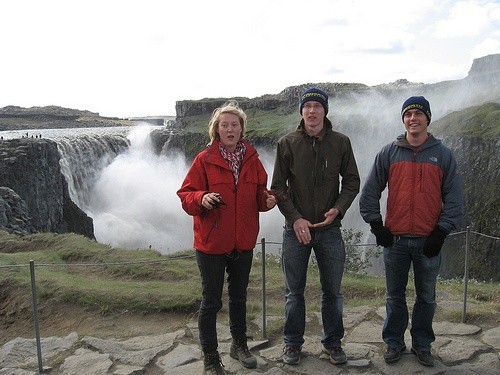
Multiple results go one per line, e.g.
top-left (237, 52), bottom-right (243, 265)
top-left (177, 105), bottom-right (276, 375)
top-left (359, 97), bottom-right (464, 366)
top-left (270, 87), bottom-right (361, 364)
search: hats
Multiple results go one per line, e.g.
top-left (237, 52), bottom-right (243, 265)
top-left (300, 88), bottom-right (329, 116)
top-left (402, 96), bottom-right (431, 125)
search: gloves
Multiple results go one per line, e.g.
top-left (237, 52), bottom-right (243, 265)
top-left (423, 226), bottom-right (447, 258)
top-left (370, 221), bottom-right (393, 247)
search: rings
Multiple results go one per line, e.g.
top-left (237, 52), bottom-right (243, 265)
top-left (302, 230), bottom-right (305, 232)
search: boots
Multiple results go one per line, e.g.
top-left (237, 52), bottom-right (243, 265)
top-left (203, 348), bottom-right (225, 375)
top-left (229, 334), bottom-right (257, 368)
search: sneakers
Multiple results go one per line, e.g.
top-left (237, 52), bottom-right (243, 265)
top-left (283, 345), bottom-right (301, 364)
top-left (384, 342), bottom-right (406, 362)
top-left (411, 346), bottom-right (434, 365)
top-left (322, 343), bottom-right (347, 364)
